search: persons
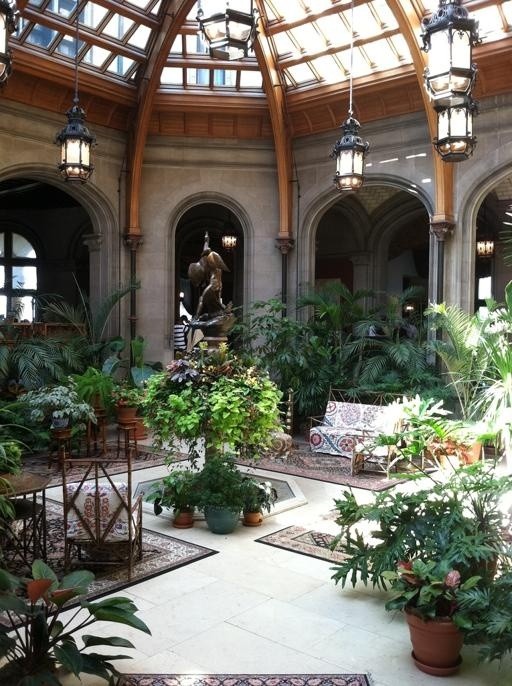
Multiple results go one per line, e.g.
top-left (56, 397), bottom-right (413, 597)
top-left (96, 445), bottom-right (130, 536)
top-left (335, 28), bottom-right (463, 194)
top-left (173, 315), bottom-right (196, 353)
top-left (368, 315), bottom-right (382, 336)
top-left (401, 312), bottom-right (420, 340)
top-left (187, 230), bottom-right (230, 319)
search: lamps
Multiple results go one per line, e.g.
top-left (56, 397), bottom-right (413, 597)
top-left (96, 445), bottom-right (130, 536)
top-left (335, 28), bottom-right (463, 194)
top-left (53, 0), bottom-right (96, 184)
top-left (197, 0), bottom-right (263, 61)
top-left (433, 103), bottom-right (478, 161)
top-left (419, 0), bottom-right (480, 110)
top-left (330, 0), bottom-right (370, 195)
top-left (220, 232), bottom-right (239, 252)
top-left (477, 237), bottom-right (495, 258)
